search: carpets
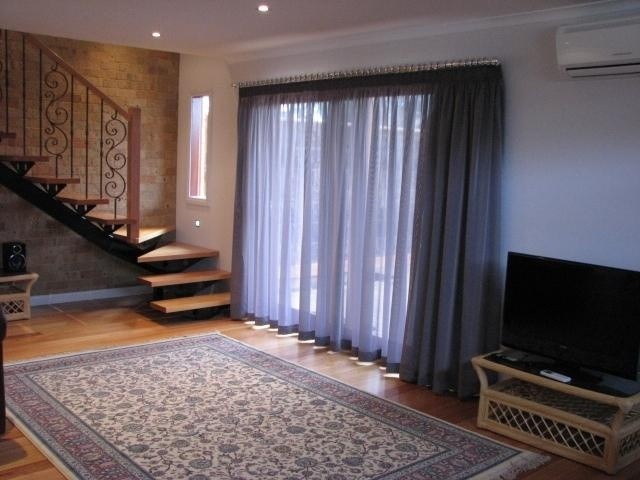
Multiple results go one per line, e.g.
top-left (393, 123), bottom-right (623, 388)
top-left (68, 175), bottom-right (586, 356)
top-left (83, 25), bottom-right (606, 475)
top-left (2, 331), bottom-right (552, 479)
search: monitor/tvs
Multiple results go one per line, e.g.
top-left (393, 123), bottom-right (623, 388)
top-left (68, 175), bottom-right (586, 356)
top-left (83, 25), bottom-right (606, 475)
top-left (501, 252), bottom-right (640, 381)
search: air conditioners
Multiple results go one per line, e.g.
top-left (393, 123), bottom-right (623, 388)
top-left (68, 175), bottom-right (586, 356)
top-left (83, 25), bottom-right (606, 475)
top-left (556, 15), bottom-right (640, 77)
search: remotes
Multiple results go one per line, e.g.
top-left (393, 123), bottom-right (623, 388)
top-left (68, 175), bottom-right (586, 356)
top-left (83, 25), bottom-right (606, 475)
top-left (540, 369), bottom-right (572, 383)
top-left (496, 353), bottom-right (519, 363)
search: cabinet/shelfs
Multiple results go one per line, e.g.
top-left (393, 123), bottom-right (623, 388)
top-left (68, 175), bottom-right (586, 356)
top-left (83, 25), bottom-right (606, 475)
top-left (470, 349), bottom-right (640, 476)
top-left (0, 271), bottom-right (39, 321)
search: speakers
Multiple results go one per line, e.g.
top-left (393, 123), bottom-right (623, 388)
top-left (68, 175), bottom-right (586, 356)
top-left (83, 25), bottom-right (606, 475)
top-left (3, 242), bottom-right (26, 275)
